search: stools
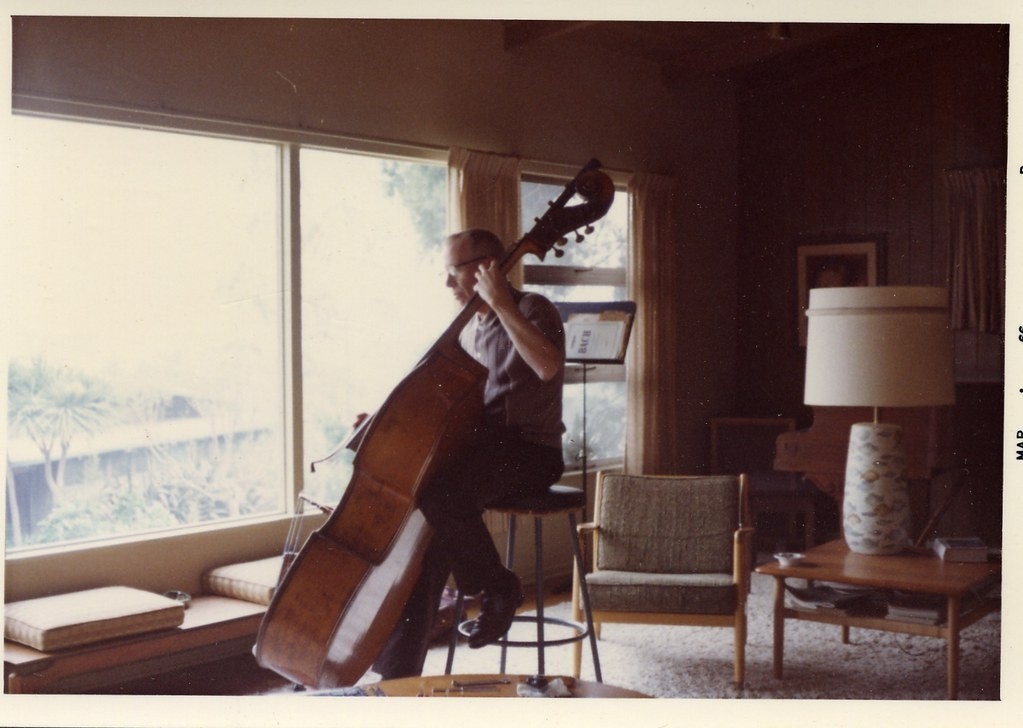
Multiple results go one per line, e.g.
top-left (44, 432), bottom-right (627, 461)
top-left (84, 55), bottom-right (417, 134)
top-left (442, 485), bottom-right (603, 683)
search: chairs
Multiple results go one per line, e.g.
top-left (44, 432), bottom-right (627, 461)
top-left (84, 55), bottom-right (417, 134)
top-left (570, 470), bottom-right (754, 698)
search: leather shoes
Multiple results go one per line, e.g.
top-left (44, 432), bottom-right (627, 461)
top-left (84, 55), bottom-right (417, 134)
top-left (468, 572), bottom-right (525, 648)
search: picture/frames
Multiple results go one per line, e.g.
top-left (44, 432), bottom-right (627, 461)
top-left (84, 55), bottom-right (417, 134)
top-left (790, 234), bottom-right (887, 352)
top-left (710, 416), bottom-right (796, 543)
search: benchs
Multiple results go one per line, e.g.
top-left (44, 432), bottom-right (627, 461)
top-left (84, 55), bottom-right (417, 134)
top-left (4, 508), bottom-right (330, 694)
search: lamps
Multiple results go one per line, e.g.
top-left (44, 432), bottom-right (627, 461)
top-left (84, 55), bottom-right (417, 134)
top-left (801, 284), bottom-right (958, 557)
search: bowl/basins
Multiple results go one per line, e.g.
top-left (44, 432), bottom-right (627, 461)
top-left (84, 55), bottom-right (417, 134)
top-left (774, 553), bottom-right (805, 566)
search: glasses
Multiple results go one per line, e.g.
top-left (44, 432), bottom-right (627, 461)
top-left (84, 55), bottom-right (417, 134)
top-left (444, 255), bottom-right (496, 277)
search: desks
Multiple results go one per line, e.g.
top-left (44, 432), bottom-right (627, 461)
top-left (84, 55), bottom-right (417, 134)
top-left (756, 533), bottom-right (1001, 701)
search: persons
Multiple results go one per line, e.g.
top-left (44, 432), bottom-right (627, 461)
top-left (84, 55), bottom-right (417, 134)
top-left (349, 230), bottom-right (566, 690)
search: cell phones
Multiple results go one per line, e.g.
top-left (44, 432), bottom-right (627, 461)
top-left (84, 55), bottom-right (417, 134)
top-left (517, 675), bottom-right (575, 688)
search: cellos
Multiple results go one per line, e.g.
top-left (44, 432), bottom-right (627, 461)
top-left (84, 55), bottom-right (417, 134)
top-left (255, 158), bottom-right (614, 691)
